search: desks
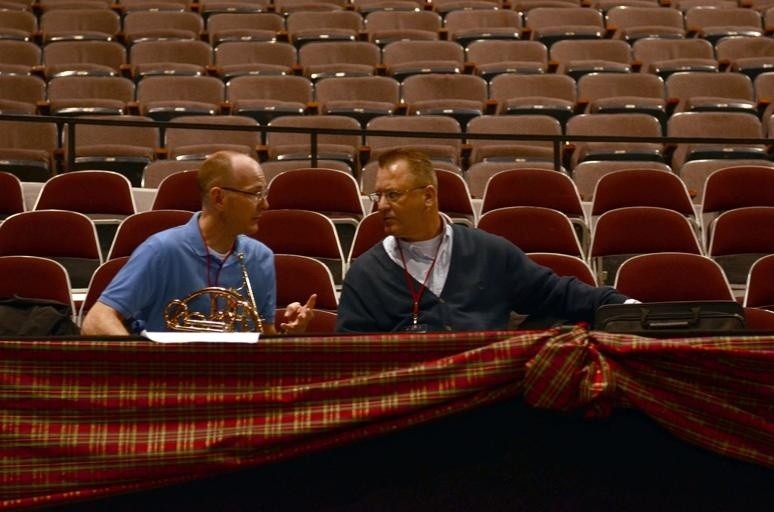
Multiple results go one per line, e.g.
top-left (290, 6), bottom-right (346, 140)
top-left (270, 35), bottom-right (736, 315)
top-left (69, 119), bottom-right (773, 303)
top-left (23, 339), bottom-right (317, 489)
top-left (0, 328), bottom-right (774, 511)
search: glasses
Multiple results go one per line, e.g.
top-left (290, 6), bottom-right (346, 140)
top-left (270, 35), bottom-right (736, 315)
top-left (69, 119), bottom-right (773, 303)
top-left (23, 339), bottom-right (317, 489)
top-left (220, 186), bottom-right (270, 201)
top-left (367, 184), bottom-right (426, 202)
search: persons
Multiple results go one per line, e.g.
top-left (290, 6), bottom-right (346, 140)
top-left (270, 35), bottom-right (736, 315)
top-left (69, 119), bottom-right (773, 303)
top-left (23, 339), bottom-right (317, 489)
top-left (79, 149), bottom-right (316, 339)
top-left (333, 149), bottom-right (643, 336)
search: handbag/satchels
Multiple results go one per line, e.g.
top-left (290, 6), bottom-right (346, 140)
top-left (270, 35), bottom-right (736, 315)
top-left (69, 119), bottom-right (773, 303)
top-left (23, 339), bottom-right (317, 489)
top-left (593, 299), bottom-right (746, 336)
